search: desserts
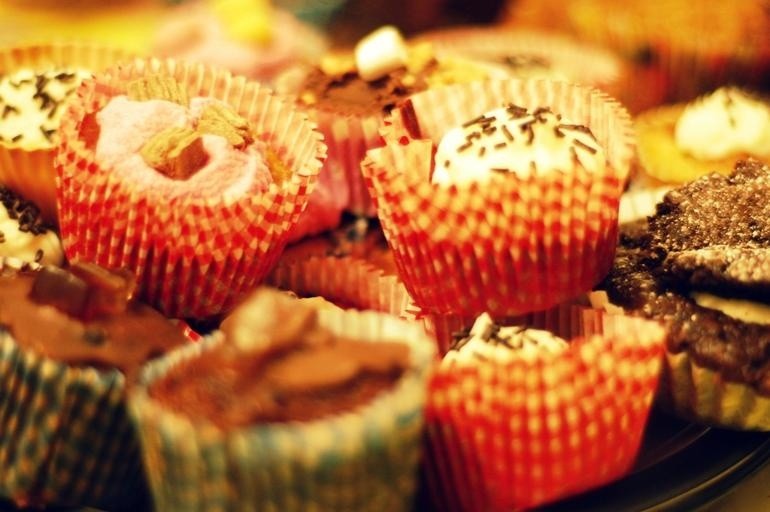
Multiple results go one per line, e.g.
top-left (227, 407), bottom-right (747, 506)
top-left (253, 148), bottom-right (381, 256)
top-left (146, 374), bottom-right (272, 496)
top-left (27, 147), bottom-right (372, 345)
top-left (0, 23), bottom-right (770, 512)
